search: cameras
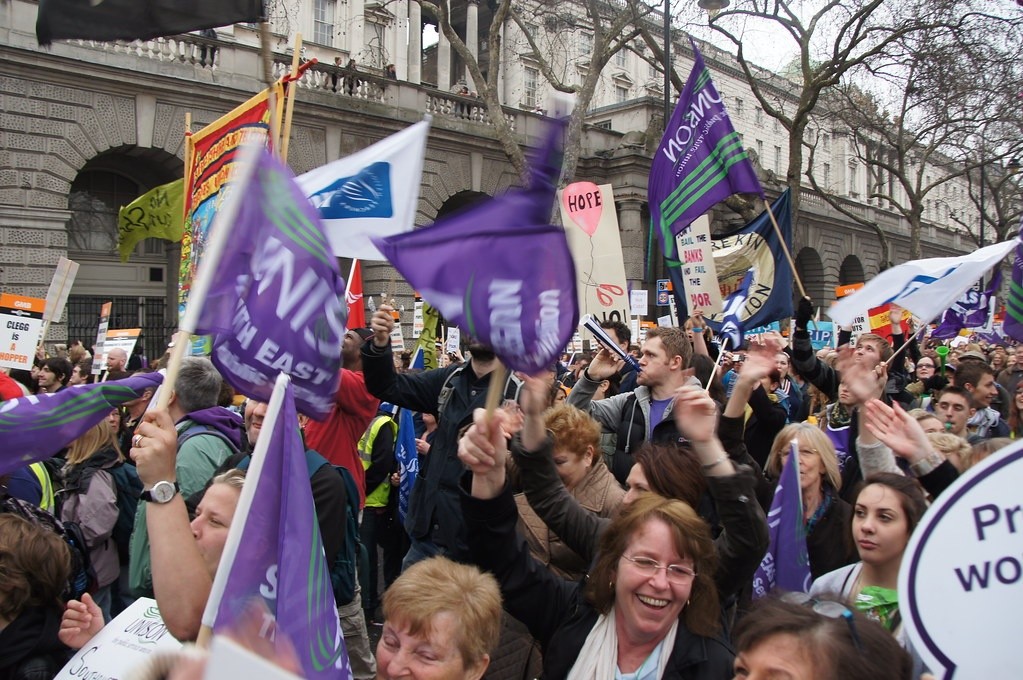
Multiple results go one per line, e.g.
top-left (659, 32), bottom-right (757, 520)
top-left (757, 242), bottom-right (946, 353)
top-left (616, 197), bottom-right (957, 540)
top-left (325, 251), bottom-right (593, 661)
top-left (730, 354), bottom-right (745, 362)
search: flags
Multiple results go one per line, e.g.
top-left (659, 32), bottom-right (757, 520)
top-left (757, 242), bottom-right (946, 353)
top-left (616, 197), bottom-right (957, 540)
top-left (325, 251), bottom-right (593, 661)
top-left (294, 121), bottom-right (429, 262)
top-left (751, 445), bottom-right (811, 599)
top-left (117, 177), bottom-right (184, 264)
top-left (1003, 217), bottom-right (1023, 345)
top-left (372, 120), bottom-right (580, 377)
top-left (930, 260), bottom-right (1004, 340)
top-left (35, 0), bottom-right (268, 48)
top-left (189, 149), bottom-right (349, 421)
top-left (824, 238), bottom-right (1021, 332)
top-left (394, 406), bottom-right (419, 518)
top-left (213, 381), bottom-right (353, 680)
top-left (0, 372), bottom-right (164, 474)
top-left (666, 187), bottom-right (793, 350)
top-left (343, 260), bottom-right (366, 329)
top-left (647, 53), bottom-right (764, 268)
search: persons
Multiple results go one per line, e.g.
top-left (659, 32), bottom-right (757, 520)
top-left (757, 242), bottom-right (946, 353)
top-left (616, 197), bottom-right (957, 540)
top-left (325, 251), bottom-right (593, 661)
top-left (0, 304), bottom-right (1023, 680)
top-left (531, 105), bottom-right (542, 115)
top-left (200, 28), bottom-right (217, 65)
top-left (325, 57), bottom-right (396, 96)
top-left (455, 86), bottom-right (477, 116)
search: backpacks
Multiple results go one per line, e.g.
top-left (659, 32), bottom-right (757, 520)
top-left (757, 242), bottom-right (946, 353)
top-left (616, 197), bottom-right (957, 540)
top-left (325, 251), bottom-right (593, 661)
top-left (91, 462), bottom-right (142, 540)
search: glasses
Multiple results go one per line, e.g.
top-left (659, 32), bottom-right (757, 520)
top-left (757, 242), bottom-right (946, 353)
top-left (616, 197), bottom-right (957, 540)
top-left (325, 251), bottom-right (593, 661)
top-left (917, 364), bottom-right (935, 368)
top-left (778, 448), bottom-right (818, 459)
top-left (1015, 390), bottom-right (1023, 394)
top-left (779, 591), bottom-right (860, 653)
top-left (928, 345), bottom-right (937, 349)
top-left (621, 554), bottom-right (700, 587)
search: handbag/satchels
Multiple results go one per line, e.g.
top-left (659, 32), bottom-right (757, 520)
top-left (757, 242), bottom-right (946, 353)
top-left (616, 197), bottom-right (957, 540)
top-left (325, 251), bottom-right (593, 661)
top-left (61, 521), bottom-right (98, 604)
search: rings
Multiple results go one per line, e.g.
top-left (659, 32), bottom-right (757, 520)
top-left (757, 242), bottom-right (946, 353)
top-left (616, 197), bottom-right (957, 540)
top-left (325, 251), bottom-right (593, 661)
top-left (134, 435), bottom-right (144, 448)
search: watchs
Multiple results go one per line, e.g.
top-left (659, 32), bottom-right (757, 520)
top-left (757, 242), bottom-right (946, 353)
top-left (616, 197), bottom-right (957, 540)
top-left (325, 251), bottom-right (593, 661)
top-left (139, 480), bottom-right (179, 504)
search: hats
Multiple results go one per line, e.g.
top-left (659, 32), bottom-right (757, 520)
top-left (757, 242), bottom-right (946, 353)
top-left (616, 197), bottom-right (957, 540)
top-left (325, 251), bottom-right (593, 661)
top-left (933, 362), bottom-right (957, 373)
top-left (350, 327), bottom-right (373, 341)
top-left (957, 350), bottom-right (986, 361)
